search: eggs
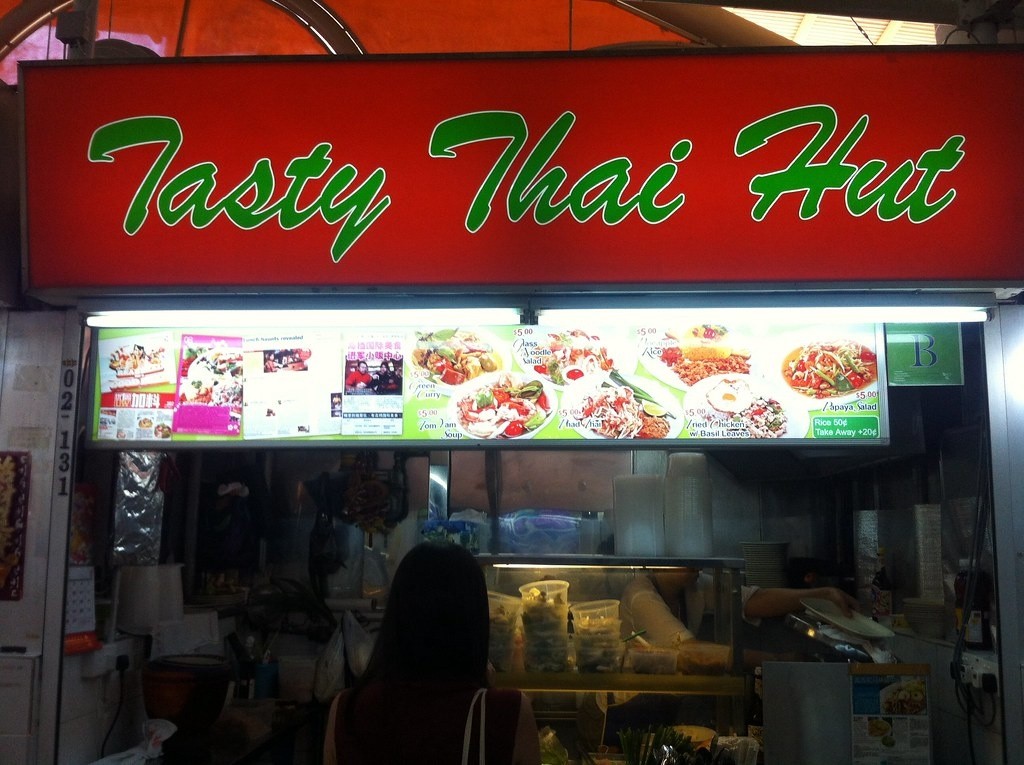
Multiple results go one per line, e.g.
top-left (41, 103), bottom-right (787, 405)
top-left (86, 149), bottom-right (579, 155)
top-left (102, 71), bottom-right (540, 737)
top-left (707, 381), bottom-right (749, 414)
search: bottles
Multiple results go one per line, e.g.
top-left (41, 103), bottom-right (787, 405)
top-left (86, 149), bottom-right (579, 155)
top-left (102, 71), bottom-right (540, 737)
top-left (747, 667), bottom-right (764, 765)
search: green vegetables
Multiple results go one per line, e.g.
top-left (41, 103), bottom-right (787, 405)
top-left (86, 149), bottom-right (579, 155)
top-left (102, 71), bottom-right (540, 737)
top-left (413, 327), bottom-right (458, 365)
top-left (503, 380), bottom-right (544, 399)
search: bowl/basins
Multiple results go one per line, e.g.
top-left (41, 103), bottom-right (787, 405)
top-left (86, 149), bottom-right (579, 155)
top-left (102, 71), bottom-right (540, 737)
top-left (447, 370), bottom-right (558, 439)
top-left (487, 580), bottom-right (729, 673)
top-left (612, 452), bottom-right (714, 558)
top-left (669, 726), bottom-right (717, 748)
top-left (401, 326), bottom-right (511, 395)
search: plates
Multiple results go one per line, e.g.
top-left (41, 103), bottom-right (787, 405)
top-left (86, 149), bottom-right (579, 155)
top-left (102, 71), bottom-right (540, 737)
top-left (561, 373), bottom-right (685, 439)
top-left (771, 332), bottom-right (877, 410)
top-left (744, 541), bottom-right (789, 589)
top-left (512, 324), bottom-right (638, 391)
top-left (798, 596), bottom-right (895, 639)
top-left (683, 373), bottom-right (811, 438)
top-left (637, 318), bottom-right (769, 391)
top-left (902, 597), bottom-right (955, 639)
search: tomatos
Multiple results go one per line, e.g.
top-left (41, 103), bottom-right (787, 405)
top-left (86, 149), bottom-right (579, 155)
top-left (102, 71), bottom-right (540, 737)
top-left (493, 389), bottom-right (549, 436)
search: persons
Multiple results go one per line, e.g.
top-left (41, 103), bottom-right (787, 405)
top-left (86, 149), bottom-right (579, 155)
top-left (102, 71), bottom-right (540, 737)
top-left (264, 352), bottom-right (279, 372)
top-left (619, 565), bottom-right (862, 671)
top-left (346, 362), bottom-right (394, 395)
top-left (323, 543), bottom-right (541, 765)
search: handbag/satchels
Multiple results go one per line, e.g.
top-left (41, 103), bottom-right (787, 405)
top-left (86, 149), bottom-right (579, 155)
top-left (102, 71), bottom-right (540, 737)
top-left (313, 612), bottom-right (345, 702)
top-left (342, 607), bottom-right (375, 676)
